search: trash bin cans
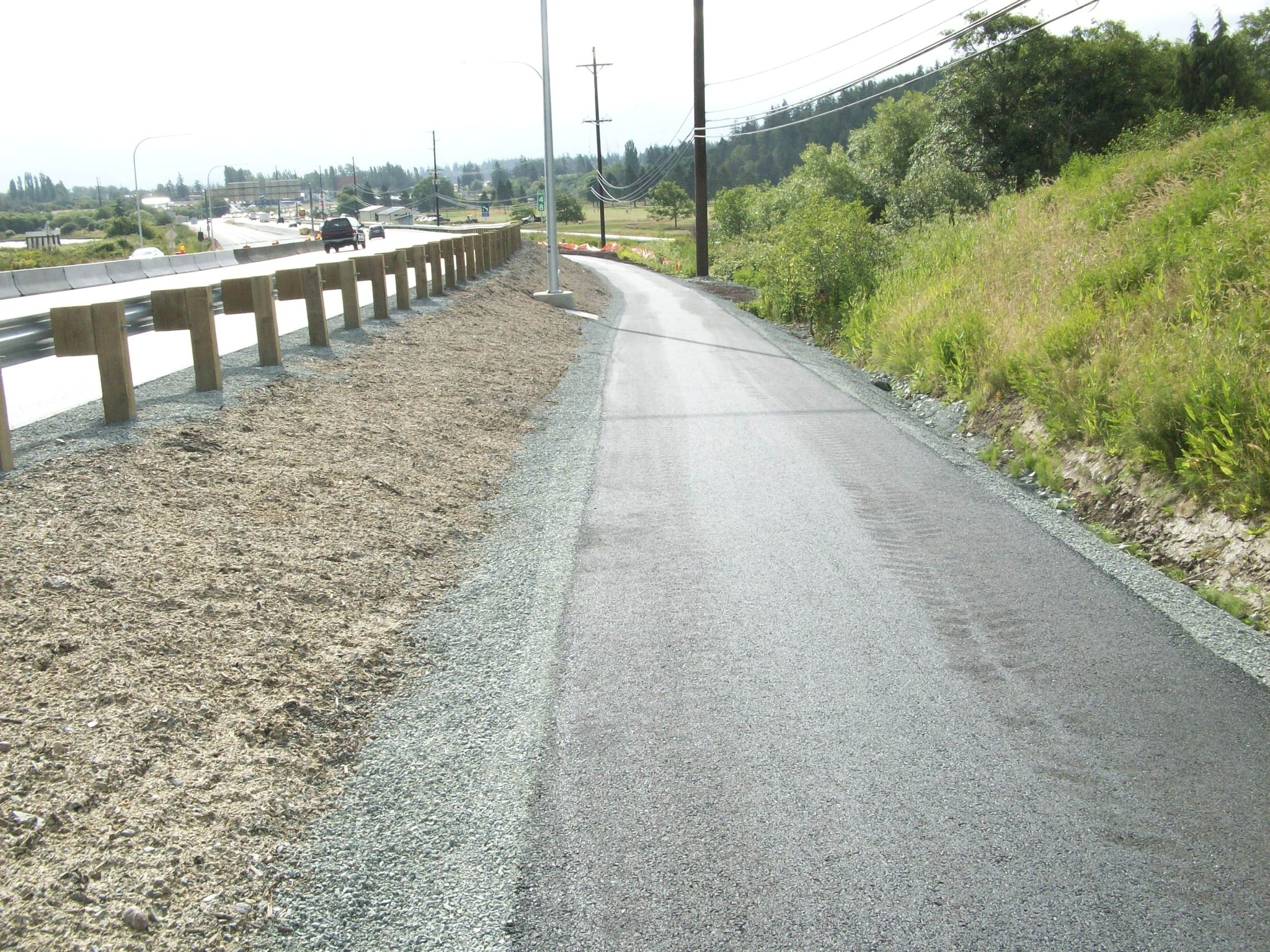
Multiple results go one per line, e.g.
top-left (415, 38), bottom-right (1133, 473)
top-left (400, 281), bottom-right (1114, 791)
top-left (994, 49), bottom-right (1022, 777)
top-left (175, 250), bottom-right (186, 254)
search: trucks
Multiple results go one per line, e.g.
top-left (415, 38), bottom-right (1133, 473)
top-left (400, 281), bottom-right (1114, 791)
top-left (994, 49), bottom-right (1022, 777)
top-left (259, 214), bottom-right (269, 222)
top-left (251, 213), bottom-right (256, 221)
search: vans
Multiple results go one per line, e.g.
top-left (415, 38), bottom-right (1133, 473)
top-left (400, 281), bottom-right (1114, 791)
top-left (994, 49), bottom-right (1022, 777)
top-left (321, 217), bottom-right (365, 253)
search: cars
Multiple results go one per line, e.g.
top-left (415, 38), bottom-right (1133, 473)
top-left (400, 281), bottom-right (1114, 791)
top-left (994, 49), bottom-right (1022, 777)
top-left (276, 217), bottom-right (284, 224)
top-left (367, 225), bottom-right (385, 240)
top-left (293, 218), bottom-right (301, 225)
top-left (289, 221), bottom-right (297, 228)
